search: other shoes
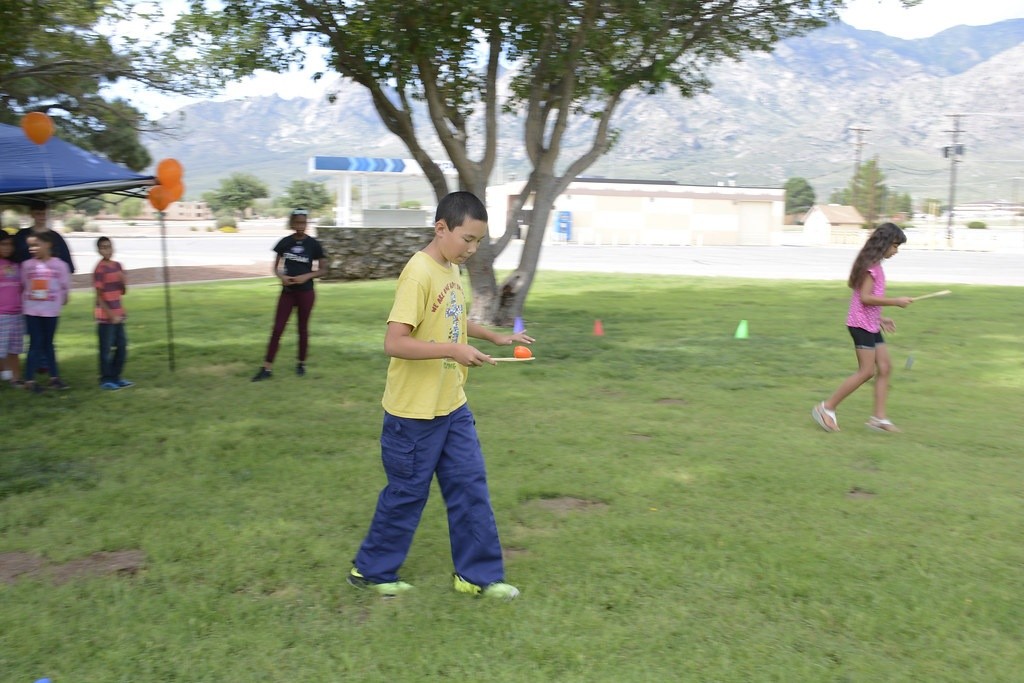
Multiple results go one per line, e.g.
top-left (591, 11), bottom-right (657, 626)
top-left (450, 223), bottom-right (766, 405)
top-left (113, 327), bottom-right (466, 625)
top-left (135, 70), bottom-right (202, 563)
top-left (9, 380), bottom-right (25, 389)
top-left (250, 366), bottom-right (273, 383)
top-left (293, 363), bottom-right (306, 377)
top-left (0, 370), bottom-right (14, 381)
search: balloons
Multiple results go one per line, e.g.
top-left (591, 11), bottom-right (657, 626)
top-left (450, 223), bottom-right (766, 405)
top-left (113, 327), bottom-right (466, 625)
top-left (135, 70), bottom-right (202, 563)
top-left (23, 112), bottom-right (51, 145)
top-left (158, 160), bottom-right (183, 187)
top-left (149, 185), bottom-right (168, 210)
top-left (167, 182), bottom-right (183, 202)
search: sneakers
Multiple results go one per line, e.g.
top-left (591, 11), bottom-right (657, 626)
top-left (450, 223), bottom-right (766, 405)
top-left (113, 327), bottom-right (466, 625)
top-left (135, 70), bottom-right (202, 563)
top-left (115, 379), bottom-right (134, 388)
top-left (99, 379), bottom-right (123, 391)
top-left (46, 378), bottom-right (70, 391)
top-left (451, 571), bottom-right (520, 599)
top-left (346, 566), bottom-right (415, 600)
top-left (26, 379), bottom-right (47, 394)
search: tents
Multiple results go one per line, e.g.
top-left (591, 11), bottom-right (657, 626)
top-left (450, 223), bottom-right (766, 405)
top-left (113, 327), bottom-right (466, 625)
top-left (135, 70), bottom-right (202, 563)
top-left (0, 123), bottom-right (175, 375)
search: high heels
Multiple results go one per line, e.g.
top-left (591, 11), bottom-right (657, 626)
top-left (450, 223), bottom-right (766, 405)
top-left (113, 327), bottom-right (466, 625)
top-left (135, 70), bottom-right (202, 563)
top-left (864, 416), bottom-right (899, 435)
top-left (811, 400), bottom-right (841, 433)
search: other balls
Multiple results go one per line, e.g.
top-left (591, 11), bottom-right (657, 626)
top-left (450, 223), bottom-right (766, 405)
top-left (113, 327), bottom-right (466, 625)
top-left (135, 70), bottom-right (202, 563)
top-left (513, 345), bottom-right (533, 357)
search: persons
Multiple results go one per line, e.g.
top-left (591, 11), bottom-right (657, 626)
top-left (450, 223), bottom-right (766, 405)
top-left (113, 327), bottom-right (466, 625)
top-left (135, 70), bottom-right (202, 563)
top-left (15, 202), bottom-right (74, 372)
top-left (346, 192), bottom-right (535, 599)
top-left (19, 233), bottom-right (71, 389)
top-left (0, 230), bottom-right (26, 387)
top-left (812, 223), bottom-right (913, 432)
top-left (91, 236), bottom-right (134, 390)
top-left (252, 209), bottom-right (327, 381)
